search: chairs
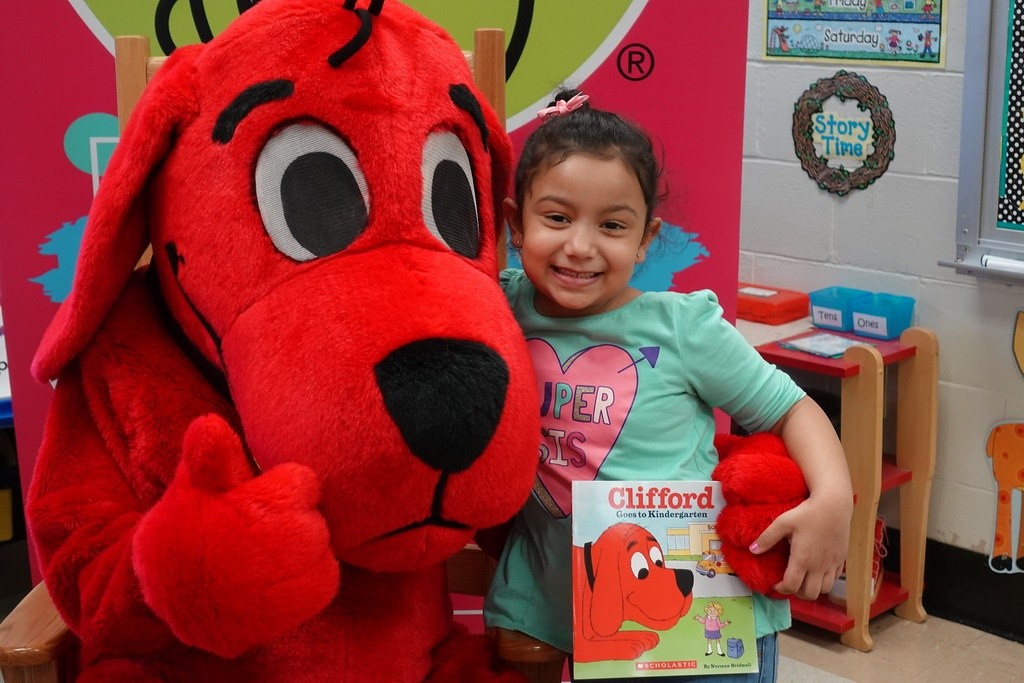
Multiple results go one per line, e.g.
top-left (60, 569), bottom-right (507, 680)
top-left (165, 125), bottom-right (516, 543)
top-left (0, 29), bottom-right (569, 683)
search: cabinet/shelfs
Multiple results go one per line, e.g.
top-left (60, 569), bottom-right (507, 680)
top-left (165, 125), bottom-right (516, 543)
top-left (736, 314), bottom-right (939, 653)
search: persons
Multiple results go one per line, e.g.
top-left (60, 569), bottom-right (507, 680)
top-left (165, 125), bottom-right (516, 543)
top-left (470, 86), bottom-right (854, 683)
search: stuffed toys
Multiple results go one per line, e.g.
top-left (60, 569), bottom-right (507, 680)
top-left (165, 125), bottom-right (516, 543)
top-left (23, 0), bottom-right (809, 683)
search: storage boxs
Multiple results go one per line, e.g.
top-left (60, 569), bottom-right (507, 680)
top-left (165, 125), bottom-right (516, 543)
top-left (738, 280), bottom-right (915, 340)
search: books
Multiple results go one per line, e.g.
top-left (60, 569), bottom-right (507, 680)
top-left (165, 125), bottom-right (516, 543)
top-left (571, 481), bottom-right (761, 679)
